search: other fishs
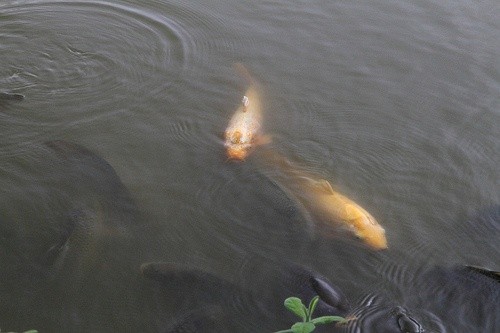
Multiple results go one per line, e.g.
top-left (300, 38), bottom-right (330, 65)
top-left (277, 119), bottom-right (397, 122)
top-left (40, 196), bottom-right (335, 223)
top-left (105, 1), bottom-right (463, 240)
top-left (46, 139), bottom-right (139, 217)
top-left (139, 259), bottom-right (269, 318)
top-left (38, 188), bottom-right (102, 291)
top-left (224, 62), bottom-right (273, 164)
top-left (266, 148), bottom-right (389, 253)
top-left (277, 258), bottom-right (500, 333)
top-left (244, 169), bottom-right (315, 242)
